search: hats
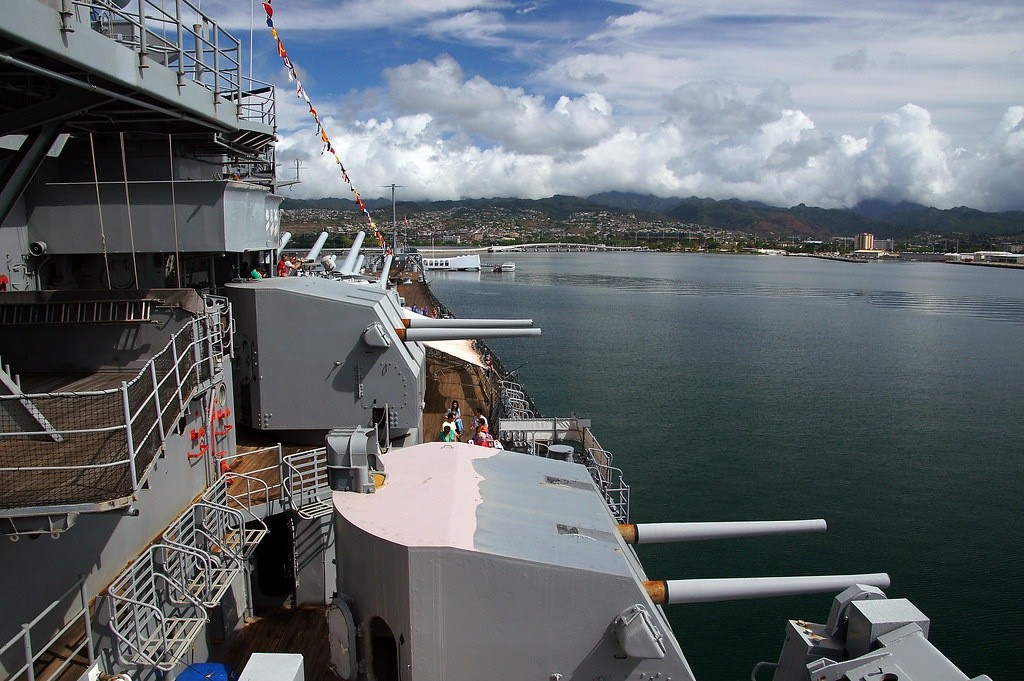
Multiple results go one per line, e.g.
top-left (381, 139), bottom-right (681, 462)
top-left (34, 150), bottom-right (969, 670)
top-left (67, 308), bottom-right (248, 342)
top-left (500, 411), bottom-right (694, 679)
top-left (476, 418), bottom-right (485, 424)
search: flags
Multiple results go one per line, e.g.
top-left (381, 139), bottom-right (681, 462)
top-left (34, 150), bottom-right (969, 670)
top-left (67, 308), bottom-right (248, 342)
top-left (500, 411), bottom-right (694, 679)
top-left (403, 216), bottom-right (408, 225)
top-left (261, 0), bottom-right (297, 82)
top-left (335, 154), bottom-right (392, 255)
top-left (297, 84), bottom-right (331, 155)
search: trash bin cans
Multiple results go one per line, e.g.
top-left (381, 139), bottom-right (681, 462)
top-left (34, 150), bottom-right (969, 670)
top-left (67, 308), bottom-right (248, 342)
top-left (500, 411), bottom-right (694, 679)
top-left (175, 662), bottom-right (232, 681)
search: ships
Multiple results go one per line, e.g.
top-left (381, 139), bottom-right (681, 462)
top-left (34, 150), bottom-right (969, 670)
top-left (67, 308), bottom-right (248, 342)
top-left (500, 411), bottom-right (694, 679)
top-left (0, 0), bottom-right (995, 681)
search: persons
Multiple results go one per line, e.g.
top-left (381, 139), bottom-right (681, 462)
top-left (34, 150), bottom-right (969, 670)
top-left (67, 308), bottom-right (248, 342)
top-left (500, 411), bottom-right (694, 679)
top-left (412, 302), bottom-right (440, 319)
top-left (243, 261), bottom-right (261, 279)
top-left (433, 400), bottom-right (463, 442)
top-left (470, 408), bottom-right (489, 447)
top-left (277, 255), bottom-right (304, 277)
